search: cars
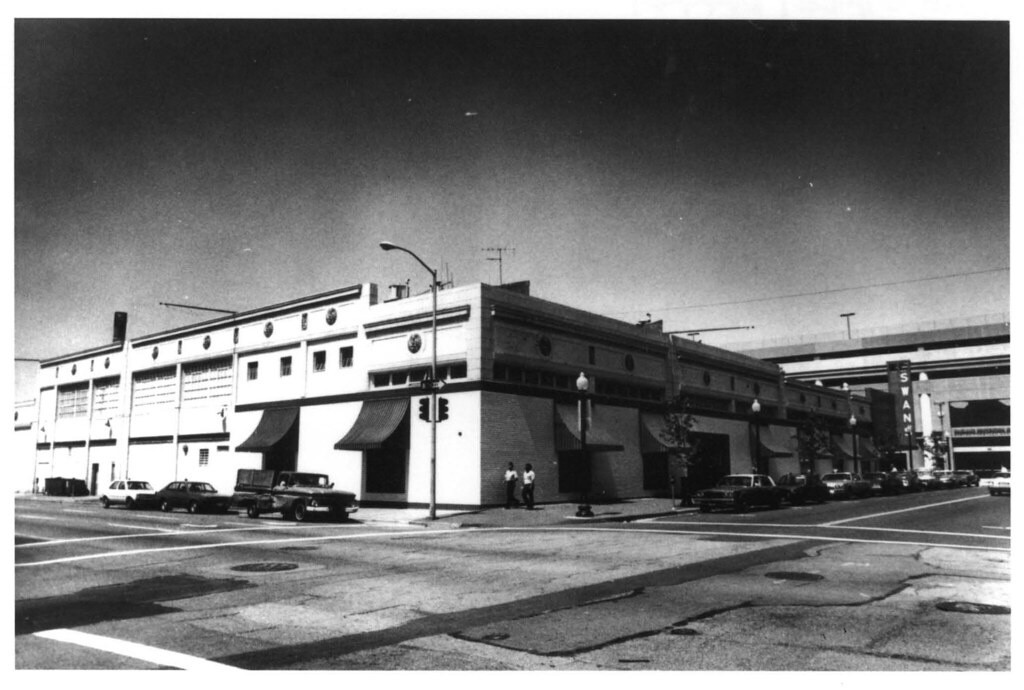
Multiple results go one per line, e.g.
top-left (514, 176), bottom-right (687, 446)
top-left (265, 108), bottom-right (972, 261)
top-left (820, 472), bottom-right (870, 499)
top-left (99, 479), bottom-right (157, 510)
top-left (987, 472), bottom-right (1010, 496)
top-left (691, 474), bottom-right (784, 515)
top-left (777, 473), bottom-right (827, 506)
top-left (154, 481), bottom-right (234, 515)
top-left (853, 466), bottom-right (980, 496)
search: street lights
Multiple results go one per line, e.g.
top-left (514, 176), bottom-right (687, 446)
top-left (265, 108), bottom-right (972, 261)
top-left (379, 242), bottom-right (451, 526)
top-left (751, 399), bottom-right (761, 474)
top-left (850, 413), bottom-right (859, 472)
top-left (574, 371), bottom-right (594, 517)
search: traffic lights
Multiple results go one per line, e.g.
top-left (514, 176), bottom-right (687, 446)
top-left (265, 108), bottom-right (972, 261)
top-left (441, 397), bottom-right (449, 420)
top-left (419, 398), bottom-right (427, 420)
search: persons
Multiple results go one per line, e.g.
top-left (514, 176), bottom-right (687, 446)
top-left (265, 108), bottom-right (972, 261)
top-left (522, 463), bottom-right (536, 510)
top-left (502, 462), bottom-right (520, 509)
top-left (1001, 464), bottom-right (1008, 472)
top-left (890, 463), bottom-right (898, 472)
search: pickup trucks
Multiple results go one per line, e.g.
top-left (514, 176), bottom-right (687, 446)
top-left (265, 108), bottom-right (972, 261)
top-left (234, 469), bottom-right (360, 522)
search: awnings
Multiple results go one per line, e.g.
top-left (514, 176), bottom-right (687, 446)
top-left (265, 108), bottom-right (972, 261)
top-left (750, 425), bottom-right (793, 458)
top-left (235, 406), bottom-right (298, 452)
top-left (640, 414), bottom-right (692, 452)
top-left (334, 397), bottom-right (409, 450)
top-left (859, 437), bottom-right (882, 458)
top-left (554, 401), bottom-right (624, 451)
top-left (831, 434), bottom-right (864, 460)
top-left (798, 431), bottom-right (833, 459)
